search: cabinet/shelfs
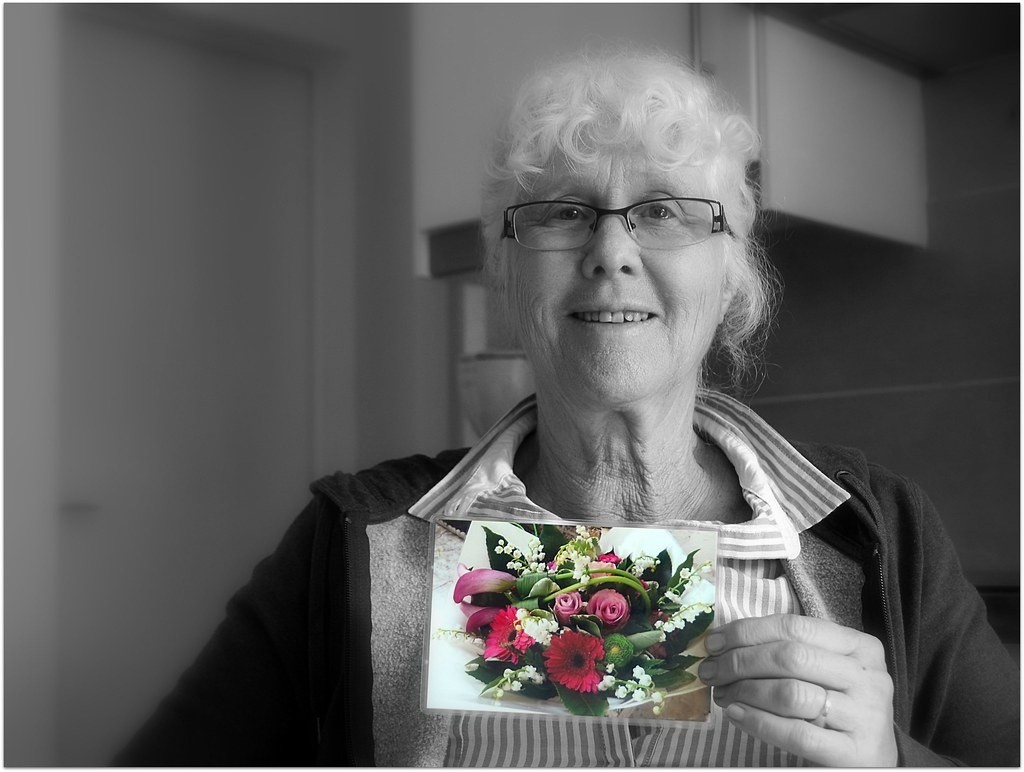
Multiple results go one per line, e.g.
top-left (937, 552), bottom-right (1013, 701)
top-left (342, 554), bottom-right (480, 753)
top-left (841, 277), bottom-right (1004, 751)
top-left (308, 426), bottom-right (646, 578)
top-left (405, 3), bottom-right (930, 275)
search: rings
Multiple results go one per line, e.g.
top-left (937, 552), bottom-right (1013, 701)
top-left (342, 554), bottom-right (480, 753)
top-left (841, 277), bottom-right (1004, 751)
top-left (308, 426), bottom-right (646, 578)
top-left (808, 689), bottom-right (832, 728)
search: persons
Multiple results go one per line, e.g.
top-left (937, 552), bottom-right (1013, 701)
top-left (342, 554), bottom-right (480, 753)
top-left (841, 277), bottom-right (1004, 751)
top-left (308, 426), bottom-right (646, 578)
top-left (110, 52), bottom-right (1021, 768)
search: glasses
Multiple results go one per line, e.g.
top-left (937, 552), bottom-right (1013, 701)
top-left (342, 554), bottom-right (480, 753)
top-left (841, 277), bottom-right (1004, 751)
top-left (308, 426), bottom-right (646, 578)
top-left (500, 197), bottom-right (738, 251)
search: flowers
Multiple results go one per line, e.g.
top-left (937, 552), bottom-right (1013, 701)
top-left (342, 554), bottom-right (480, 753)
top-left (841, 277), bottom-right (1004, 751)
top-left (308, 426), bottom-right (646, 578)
top-left (439, 525), bottom-right (716, 720)
